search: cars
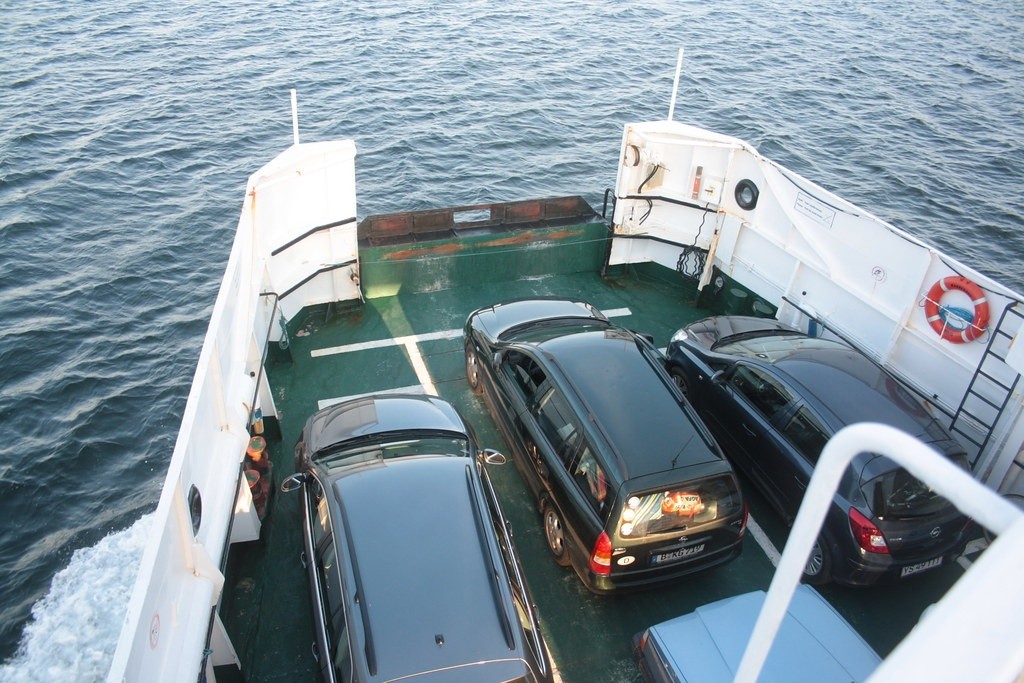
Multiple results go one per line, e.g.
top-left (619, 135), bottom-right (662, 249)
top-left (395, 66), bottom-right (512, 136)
top-left (281, 394), bottom-right (553, 683)
top-left (668, 316), bottom-right (974, 584)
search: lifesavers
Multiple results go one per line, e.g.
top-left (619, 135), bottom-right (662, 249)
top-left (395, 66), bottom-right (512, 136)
top-left (924, 274), bottom-right (990, 345)
top-left (734, 178), bottom-right (759, 211)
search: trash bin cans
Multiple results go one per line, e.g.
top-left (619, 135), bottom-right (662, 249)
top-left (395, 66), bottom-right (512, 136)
top-left (246, 436), bottom-right (270, 474)
top-left (243, 469), bottom-right (266, 518)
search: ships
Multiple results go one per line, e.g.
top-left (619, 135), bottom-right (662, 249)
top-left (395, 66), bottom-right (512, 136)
top-left (103, 46), bottom-right (1023, 682)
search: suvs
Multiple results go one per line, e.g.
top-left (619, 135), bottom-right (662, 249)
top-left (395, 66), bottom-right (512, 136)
top-left (462, 301), bottom-right (749, 593)
